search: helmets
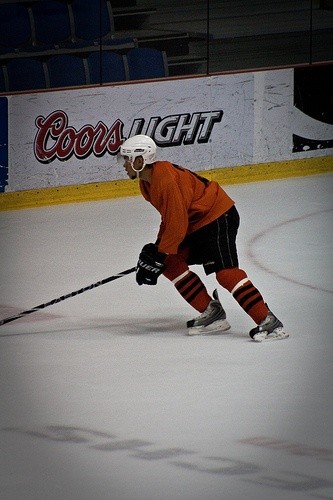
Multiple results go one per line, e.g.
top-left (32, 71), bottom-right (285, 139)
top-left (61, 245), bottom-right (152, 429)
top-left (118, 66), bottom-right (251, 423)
top-left (120, 134), bottom-right (158, 172)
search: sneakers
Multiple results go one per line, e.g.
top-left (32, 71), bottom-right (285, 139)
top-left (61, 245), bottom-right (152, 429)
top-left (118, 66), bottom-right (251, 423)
top-left (187, 288), bottom-right (231, 335)
top-left (248, 303), bottom-right (289, 342)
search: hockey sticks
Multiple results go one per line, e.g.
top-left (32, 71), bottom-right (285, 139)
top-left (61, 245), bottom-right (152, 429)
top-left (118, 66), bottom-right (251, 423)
top-left (0, 265), bottom-right (138, 325)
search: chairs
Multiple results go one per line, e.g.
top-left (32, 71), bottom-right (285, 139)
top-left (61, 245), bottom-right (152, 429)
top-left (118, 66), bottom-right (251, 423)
top-left (1, 59), bottom-right (49, 91)
top-left (0, 1), bottom-right (55, 62)
top-left (44, 54), bottom-right (90, 89)
top-left (69, 2), bottom-right (139, 54)
top-left (122, 45), bottom-right (171, 83)
top-left (28, 2), bottom-right (100, 56)
top-left (83, 50), bottom-right (129, 82)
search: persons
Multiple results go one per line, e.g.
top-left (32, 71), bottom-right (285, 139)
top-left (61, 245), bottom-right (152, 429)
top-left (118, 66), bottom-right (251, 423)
top-left (118, 133), bottom-right (287, 344)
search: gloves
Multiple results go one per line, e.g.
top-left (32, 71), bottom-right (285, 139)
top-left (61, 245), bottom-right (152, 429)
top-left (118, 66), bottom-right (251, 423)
top-left (135, 242), bottom-right (167, 286)
